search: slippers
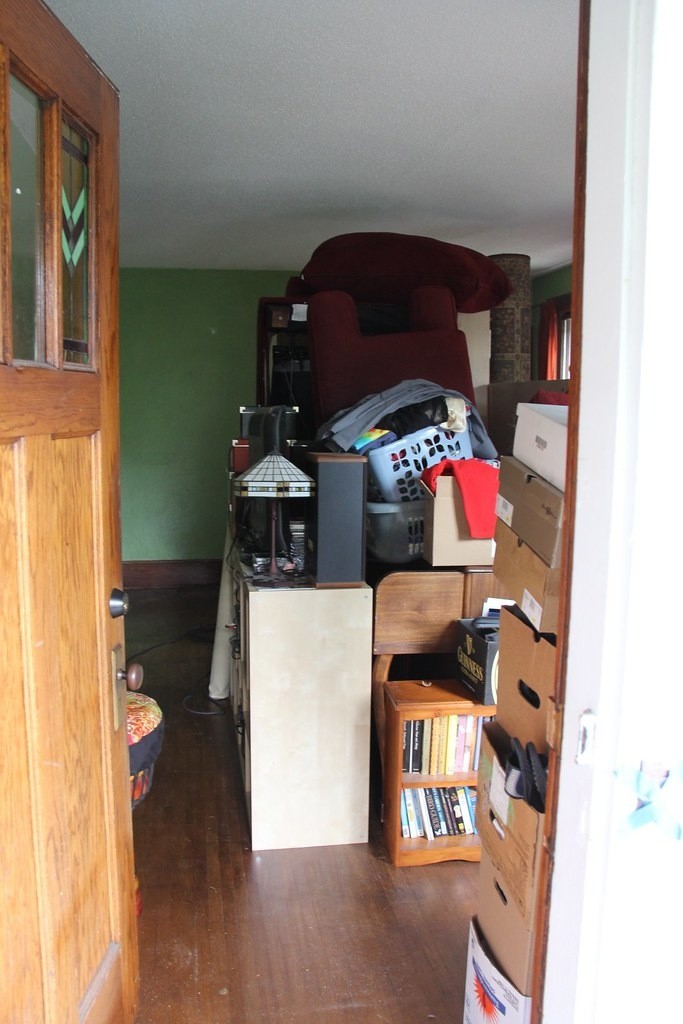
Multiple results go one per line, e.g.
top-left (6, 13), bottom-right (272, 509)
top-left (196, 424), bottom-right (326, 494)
top-left (504, 736), bottom-right (549, 810)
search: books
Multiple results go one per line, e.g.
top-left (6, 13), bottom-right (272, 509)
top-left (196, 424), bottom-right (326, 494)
top-left (401, 715), bottom-right (497, 843)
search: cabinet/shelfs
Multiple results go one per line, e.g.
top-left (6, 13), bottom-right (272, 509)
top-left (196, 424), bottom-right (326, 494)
top-left (380, 681), bottom-right (496, 868)
top-left (226, 547), bottom-right (374, 852)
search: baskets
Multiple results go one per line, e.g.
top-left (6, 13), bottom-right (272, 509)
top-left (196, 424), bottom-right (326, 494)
top-left (365, 412), bottom-right (473, 503)
top-left (367, 501), bottom-right (425, 562)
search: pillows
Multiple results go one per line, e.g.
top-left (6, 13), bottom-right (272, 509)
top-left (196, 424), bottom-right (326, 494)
top-left (299, 231), bottom-right (514, 315)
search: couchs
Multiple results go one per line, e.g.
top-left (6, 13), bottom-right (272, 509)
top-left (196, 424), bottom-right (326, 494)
top-left (304, 282), bottom-right (476, 432)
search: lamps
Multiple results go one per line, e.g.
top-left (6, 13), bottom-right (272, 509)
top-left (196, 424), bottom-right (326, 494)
top-left (233, 444), bottom-right (316, 588)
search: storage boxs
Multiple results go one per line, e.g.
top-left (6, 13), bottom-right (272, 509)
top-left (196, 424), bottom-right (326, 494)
top-left (366, 408), bottom-right (473, 502)
top-left (364, 500), bottom-right (427, 565)
top-left (417, 403), bottom-right (571, 1024)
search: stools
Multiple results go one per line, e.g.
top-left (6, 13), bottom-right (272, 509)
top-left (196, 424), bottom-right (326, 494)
top-left (124, 689), bottom-right (164, 812)
